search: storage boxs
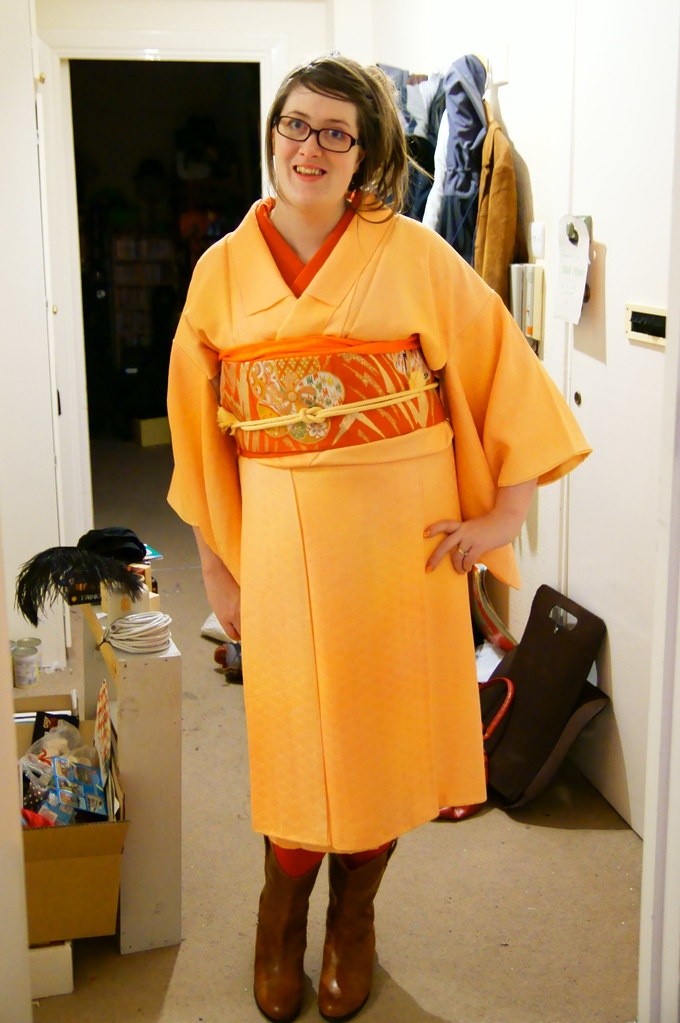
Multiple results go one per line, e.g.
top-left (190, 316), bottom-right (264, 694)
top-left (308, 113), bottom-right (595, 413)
top-left (17, 721), bottom-right (131, 945)
top-left (27, 940), bottom-right (74, 1000)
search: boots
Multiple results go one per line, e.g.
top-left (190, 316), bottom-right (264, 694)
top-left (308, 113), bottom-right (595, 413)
top-left (253, 836), bottom-right (322, 1023)
top-left (319, 839), bottom-right (398, 1023)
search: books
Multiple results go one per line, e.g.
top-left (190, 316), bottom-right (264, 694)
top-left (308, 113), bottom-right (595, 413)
top-left (472, 562), bottom-right (518, 651)
top-left (111, 239), bottom-right (174, 348)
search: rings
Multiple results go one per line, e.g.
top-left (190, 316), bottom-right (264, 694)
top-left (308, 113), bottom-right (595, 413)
top-left (457, 547), bottom-right (469, 557)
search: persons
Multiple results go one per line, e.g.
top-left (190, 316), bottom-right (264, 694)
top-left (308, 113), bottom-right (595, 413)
top-left (164, 56), bottom-right (592, 1023)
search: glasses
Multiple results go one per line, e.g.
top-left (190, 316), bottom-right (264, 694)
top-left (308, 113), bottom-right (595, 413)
top-left (270, 114), bottom-right (363, 153)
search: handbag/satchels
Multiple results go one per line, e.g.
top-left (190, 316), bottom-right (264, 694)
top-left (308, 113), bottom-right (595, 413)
top-left (434, 678), bottom-right (514, 821)
